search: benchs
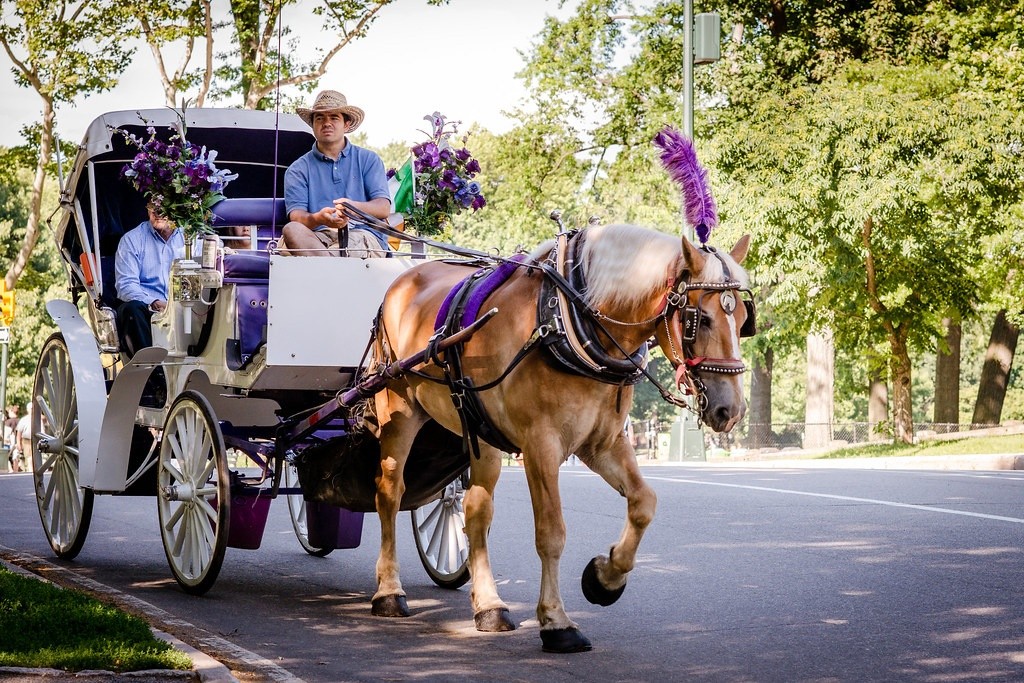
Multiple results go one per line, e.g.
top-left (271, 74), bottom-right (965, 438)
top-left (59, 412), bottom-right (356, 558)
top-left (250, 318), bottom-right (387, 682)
top-left (207, 196), bottom-right (293, 358)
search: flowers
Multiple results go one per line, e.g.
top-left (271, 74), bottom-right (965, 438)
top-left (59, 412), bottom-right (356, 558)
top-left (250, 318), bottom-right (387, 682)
top-left (402, 111), bottom-right (486, 244)
top-left (103, 98), bottom-right (239, 241)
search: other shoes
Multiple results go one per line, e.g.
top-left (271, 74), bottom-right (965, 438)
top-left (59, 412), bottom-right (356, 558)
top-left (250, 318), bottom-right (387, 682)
top-left (151, 386), bottom-right (166, 407)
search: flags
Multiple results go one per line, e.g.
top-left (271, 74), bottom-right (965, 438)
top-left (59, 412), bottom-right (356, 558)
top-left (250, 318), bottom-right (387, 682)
top-left (388, 156), bottom-right (416, 251)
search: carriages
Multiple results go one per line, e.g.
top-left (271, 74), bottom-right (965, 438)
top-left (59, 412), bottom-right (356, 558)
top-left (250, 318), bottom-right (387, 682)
top-left (32, 107), bottom-right (762, 656)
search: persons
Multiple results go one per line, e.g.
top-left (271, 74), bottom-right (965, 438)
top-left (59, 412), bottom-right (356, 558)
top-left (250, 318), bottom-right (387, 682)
top-left (0, 401), bottom-right (56, 473)
top-left (277, 90), bottom-right (391, 259)
top-left (115, 196), bottom-right (251, 376)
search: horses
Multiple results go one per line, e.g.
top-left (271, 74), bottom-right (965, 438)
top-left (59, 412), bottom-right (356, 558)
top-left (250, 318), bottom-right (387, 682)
top-left (370, 223), bottom-right (751, 654)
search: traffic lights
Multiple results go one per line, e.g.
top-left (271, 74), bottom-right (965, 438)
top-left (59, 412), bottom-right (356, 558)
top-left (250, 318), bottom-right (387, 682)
top-left (0, 279), bottom-right (15, 323)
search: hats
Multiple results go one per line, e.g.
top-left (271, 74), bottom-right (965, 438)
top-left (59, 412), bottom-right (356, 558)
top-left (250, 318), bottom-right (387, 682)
top-left (295, 90), bottom-right (366, 134)
